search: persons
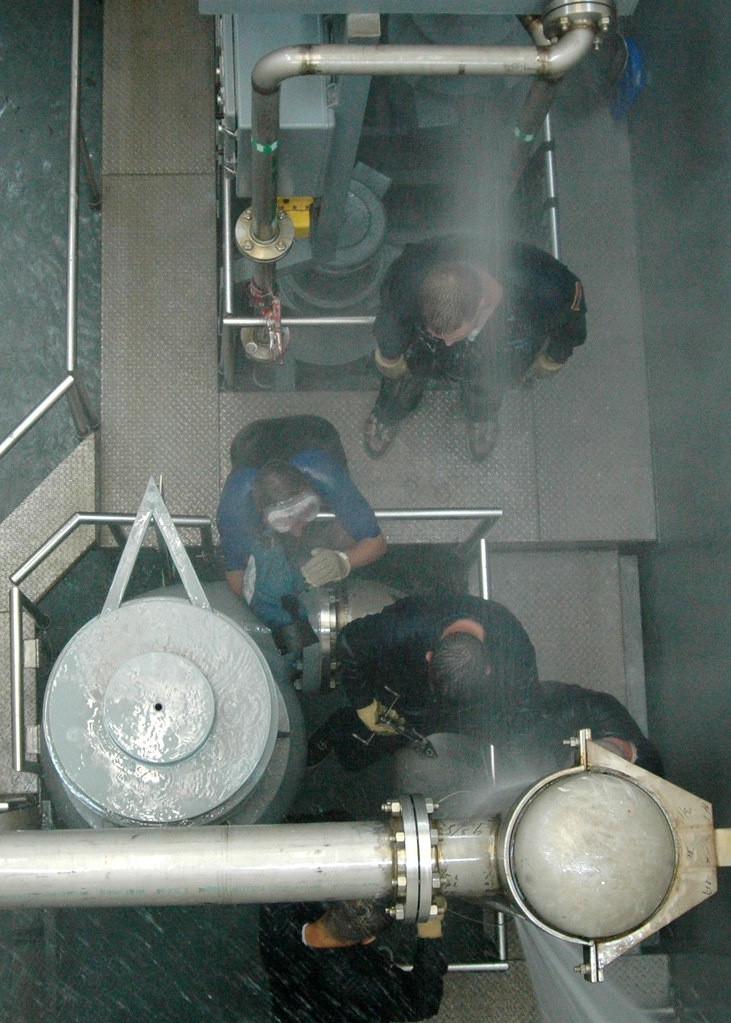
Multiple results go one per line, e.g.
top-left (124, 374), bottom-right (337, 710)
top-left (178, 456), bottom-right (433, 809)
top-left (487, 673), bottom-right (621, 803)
top-left (215, 446), bottom-right (667, 1023)
top-left (362, 234), bottom-right (589, 462)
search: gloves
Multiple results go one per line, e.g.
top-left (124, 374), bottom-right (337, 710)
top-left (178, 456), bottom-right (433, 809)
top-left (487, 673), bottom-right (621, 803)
top-left (374, 348), bottom-right (411, 381)
top-left (526, 352), bottom-right (563, 382)
top-left (416, 896), bottom-right (448, 938)
top-left (357, 698), bottom-right (406, 736)
top-left (301, 548), bottom-right (350, 586)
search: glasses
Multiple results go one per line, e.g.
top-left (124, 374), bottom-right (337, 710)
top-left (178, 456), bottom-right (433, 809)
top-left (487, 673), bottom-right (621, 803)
top-left (262, 493), bottom-right (321, 532)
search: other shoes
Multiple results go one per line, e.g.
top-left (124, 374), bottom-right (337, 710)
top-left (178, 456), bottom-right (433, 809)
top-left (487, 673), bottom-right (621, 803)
top-left (468, 418), bottom-right (498, 459)
top-left (306, 723), bottom-right (332, 770)
top-left (363, 411), bottom-right (399, 459)
